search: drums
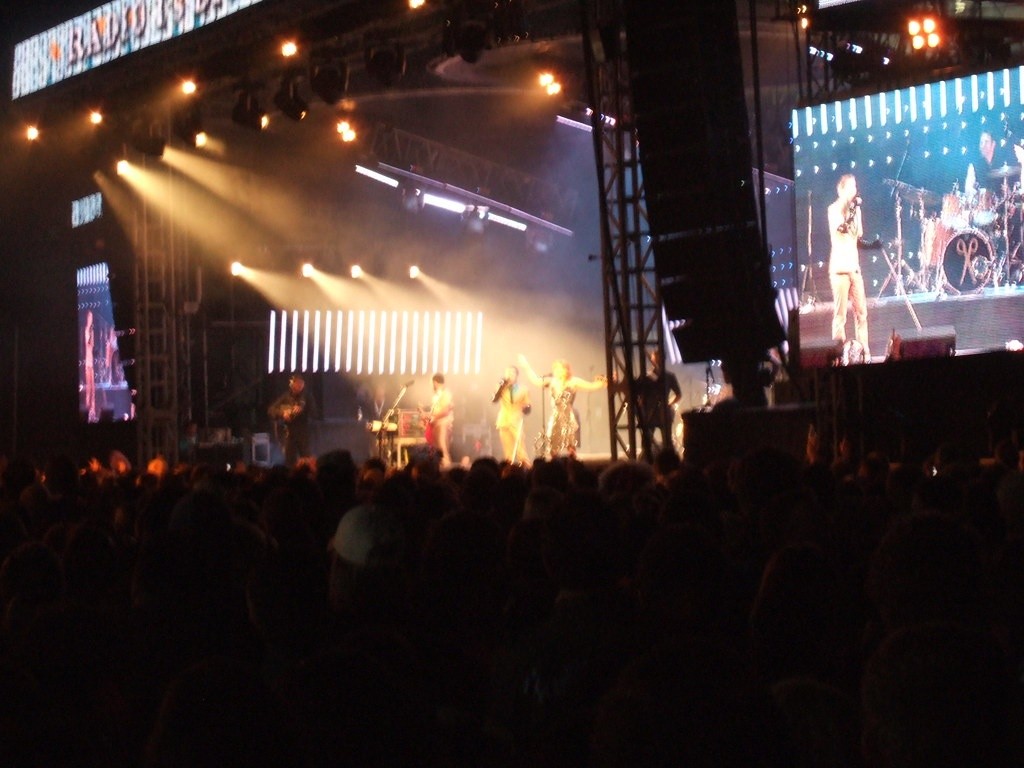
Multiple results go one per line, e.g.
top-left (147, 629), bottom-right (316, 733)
top-left (939, 228), bottom-right (993, 295)
top-left (968, 187), bottom-right (998, 226)
top-left (941, 190), bottom-right (970, 226)
top-left (920, 216), bottom-right (966, 268)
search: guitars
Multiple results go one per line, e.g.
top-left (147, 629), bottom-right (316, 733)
top-left (418, 404), bottom-right (433, 444)
top-left (373, 379), bottom-right (415, 438)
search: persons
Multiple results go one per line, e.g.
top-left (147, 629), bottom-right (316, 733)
top-left (84, 311), bottom-right (122, 420)
top-left (519, 355), bottom-right (618, 459)
top-left (421, 376), bottom-right (455, 467)
top-left (827, 174), bottom-right (873, 364)
top-left (268, 378), bottom-right (317, 469)
top-left (492, 366), bottom-right (531, 464)
top-left (2, 421), bottom-right (1024, 768)
top-left (636, 352), bottom-right (680, 450)
top-left (364, 384), bottom-right (394, 457)
top-left (965, 131), bottom-right (1012, 195)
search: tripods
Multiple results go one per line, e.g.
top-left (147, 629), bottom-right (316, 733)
top-left (878, 135), bottom-right (927, 300)
top-left (991, 175), bottom-right (1024, 288)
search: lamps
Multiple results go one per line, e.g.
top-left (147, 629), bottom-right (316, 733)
top-left (176, 117), bottom-right (203, 148)
top-left (130, 133), bottom-right (167, 155)
top-left (309, 46), bottom-right (350, 107)
top-left (232, 82), bottom-right (271, 132)
top-left (273, 68), bottom-right (309, 120)
top-left (444, 20), bottom-right (493, 67)
top-left (364, 40), bottom-right (406, 88)
top-left (355, 164), bottom-right (527, 233)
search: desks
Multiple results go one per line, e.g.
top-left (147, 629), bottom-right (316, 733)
top-left (392, 437), bottom-right (426, 470)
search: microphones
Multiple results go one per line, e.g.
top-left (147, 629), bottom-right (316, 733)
top-left (501, 379), bottom-right (509, 387)
top-left (539, 374), bottom-right (554, 379)
top-left (400, 379), bottom-right (415, 389)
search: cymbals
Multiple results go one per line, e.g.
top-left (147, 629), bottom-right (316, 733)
top-left (882, 178), bottom-right (941, 205)
top-left (986, 165), bottom-right (1021, 177)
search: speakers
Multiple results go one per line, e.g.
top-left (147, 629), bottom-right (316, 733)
top-left (887, 326), bottom-right (956, 360)
top-left (626, 0), bottom-right (786, 363)
top-left (796, 302), bottom-right (843, 367)
